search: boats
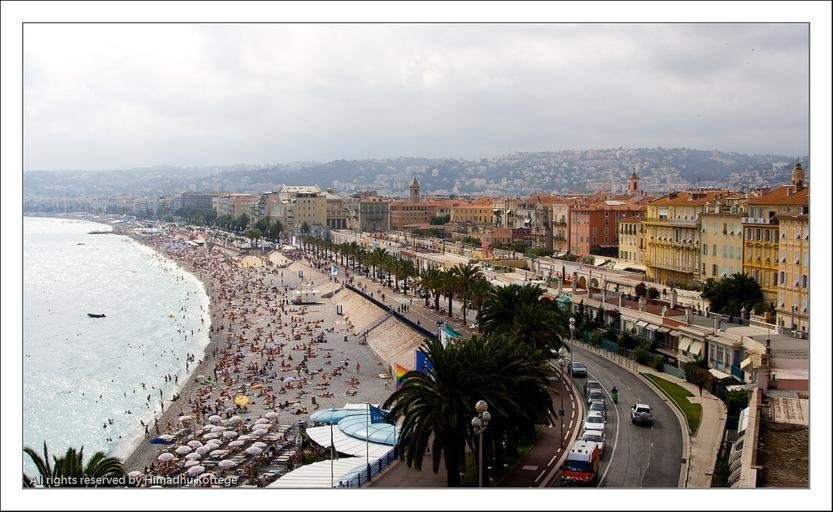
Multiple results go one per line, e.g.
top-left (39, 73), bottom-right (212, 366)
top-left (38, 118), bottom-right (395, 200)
top-left (87, 313), bottom-right (106, 318)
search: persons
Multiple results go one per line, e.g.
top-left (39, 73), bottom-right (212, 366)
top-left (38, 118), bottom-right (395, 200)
top-left (103, 232), bottom-right (445, 487)
top-left (611, 385), bottom-right (617, 394)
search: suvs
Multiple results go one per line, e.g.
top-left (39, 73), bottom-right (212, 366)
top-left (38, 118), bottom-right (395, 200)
top-left (631, 403), bottom-right (653, 426)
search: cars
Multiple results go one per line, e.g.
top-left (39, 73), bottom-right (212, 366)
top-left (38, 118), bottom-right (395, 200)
top-left (348, 260), bottom-right (369, 273)
top-left (400, 283), bottom-right (410, 290)
top-left (544, 344), bottom-right (562, 359)
top-left (376, 267), bottom-right (394, 282)
top-left (416, 289), bottom-right (431, 298)
top-left (567, 361), bottom-right (588, 378)
top-left (580, 379), bottom-right (607, 454)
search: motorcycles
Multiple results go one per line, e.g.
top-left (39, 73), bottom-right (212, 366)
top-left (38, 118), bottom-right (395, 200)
top-left (612, 393), bottom-right (618, 403)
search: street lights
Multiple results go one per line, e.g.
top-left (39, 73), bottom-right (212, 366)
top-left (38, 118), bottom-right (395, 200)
top-left (471, 400), bottom-right (494, 487)
top-left (558, 317), bottom-right (575, 448)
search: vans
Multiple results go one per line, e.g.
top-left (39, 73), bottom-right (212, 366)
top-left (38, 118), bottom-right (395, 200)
top-left (559, 439), bottom-right (601, 487)
